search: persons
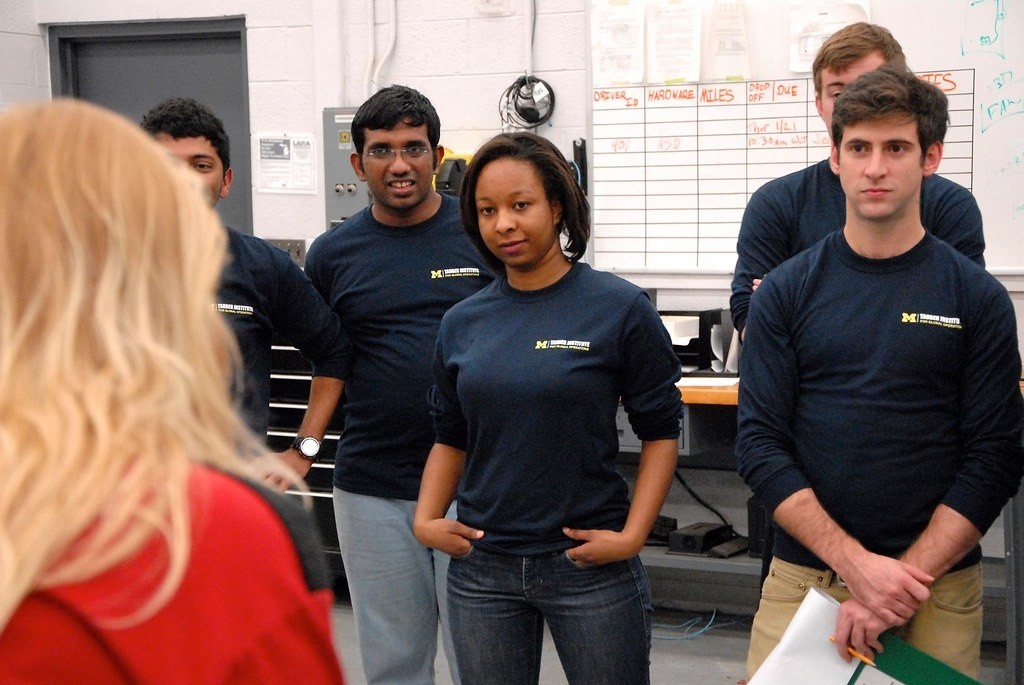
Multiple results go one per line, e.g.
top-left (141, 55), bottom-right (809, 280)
top-left (730, 22), bottom-right (987, 586)
top-left (411, 131), bottom-right (687, 685)
top-left (0, 98), bottom-right (348, 685)
top-left (303, 82), bottom-right (503, 685)
top-left (139, 99), bottom-right (347, 494)
top-left (735, 63), bottom-right (1023, 679)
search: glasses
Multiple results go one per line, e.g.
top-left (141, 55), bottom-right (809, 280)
top-left (361, 145), bottom-right (435, 159)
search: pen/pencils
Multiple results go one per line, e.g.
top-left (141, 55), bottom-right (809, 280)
top-left (829, 636), bottom-right (877, 667)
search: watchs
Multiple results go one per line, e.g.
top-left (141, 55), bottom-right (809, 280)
top-left (291, 436), bottom-right (324, 464)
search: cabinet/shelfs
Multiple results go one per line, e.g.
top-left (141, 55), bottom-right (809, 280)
top-left (598, 370), bottom-right (1024, 685)
